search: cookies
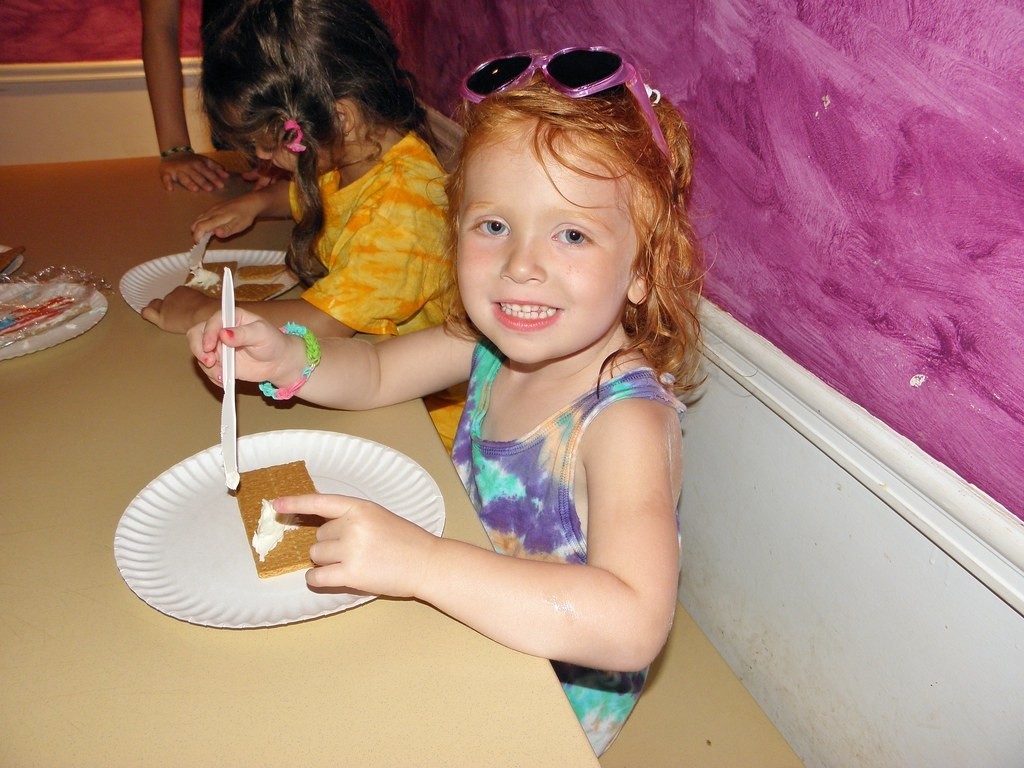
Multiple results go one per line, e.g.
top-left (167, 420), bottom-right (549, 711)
top-left (235, 460), bottom-right (330, 578)
top-left (185, 260), bottom-right (289, 301)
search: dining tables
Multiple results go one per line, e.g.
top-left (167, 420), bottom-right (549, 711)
top-left (0, 152), bottom-right (605, 767)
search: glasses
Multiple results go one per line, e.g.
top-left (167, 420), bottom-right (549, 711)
top-left (459, 46), bottom-right (672, 170)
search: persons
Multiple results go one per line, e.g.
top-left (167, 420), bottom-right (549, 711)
top-left (140, 0), bottom-right (470, 457)
top-left (185, 53), bottom-right (710, 759)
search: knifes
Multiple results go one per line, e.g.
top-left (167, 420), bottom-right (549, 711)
top-left (222, 265), bottom-right (240, 490)
top-left (189, 231), bottom-right (214, 265)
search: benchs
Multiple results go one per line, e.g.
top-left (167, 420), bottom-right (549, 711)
top-left (586, 604), bottom-right (810, 768)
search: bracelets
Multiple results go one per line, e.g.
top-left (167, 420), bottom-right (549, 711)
top-left (161, 144), bottom-right (194, 159)
top-left (258, 321), bottom-right (321, 401)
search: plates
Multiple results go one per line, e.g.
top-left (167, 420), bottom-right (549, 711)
top-left (0, 245), bottom-right (24, 276)
top-left (113, 429), bottom-right (445, 629)
top-left (0, 283), bottom-right (108, 360)
top-left (119, 249), bottom-right (299, 315)
